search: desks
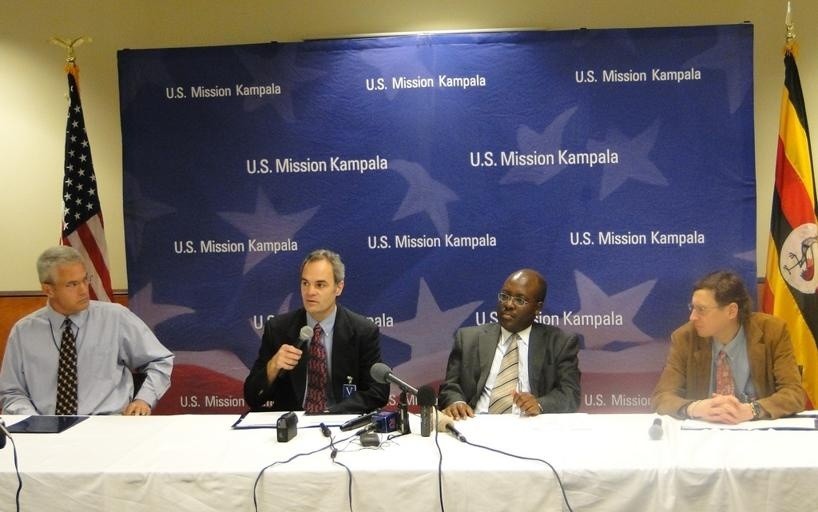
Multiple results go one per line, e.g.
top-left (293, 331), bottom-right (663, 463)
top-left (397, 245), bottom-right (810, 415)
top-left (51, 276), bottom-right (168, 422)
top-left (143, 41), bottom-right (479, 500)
top-left (0, 408), bottom-right (818, 510)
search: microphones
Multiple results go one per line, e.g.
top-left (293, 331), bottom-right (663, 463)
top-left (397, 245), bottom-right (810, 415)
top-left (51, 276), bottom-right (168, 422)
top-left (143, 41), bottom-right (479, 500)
top-left (356, 410), bottom-right (400, 435)
top-left (432, 406), bottom-right (467, 442)
top-left (340, 407), bottom-right (383, 431)
top-left (417, 384), bottom-right (436, 437)
top-left (649, 416), bottom-right (663, 440)
top-left (295, 326), bottom-right (314, 350)
top-left (370, 363), bottom-right (416, 395)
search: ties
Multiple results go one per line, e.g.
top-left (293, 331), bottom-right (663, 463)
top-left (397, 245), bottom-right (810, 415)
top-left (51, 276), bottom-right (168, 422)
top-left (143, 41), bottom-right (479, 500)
top-left (489, 334), bottom-right (519, 415)
top-left (305, 324), bottom-right (331, 416)
top-left (55, 319), bottom-right (79, 416)
top-left (715, 350), bottom-right (736, 399)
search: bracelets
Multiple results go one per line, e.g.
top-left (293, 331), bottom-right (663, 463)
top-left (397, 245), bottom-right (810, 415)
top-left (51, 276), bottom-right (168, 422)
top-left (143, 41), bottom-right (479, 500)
top-left (749, 399), bottom-right (761, 419)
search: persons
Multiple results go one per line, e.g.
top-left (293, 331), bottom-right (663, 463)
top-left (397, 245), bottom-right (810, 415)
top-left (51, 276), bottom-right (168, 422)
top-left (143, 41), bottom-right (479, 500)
top-left (647, 269), bottom-right (806, 427)
top-left (240, 246), bottom-right (391, 414)
top-left (434, 267), bottom-right (582, 419)
top-left (0, 244), bottom-right (176, 417)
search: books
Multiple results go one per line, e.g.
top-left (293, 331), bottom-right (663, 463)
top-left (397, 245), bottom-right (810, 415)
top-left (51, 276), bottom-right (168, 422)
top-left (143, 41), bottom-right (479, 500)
top-left (231, 410), bottom-right (363, 428)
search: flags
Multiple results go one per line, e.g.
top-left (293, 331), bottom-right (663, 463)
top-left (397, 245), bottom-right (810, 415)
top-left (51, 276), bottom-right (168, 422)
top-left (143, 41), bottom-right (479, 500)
top-left (48, 34), bottom-right (115, 306)
top-left (760, 1), bottom-right (817, 412)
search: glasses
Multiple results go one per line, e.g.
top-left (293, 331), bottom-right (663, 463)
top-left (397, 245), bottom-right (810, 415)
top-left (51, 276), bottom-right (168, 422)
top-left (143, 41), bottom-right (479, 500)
top-left (687, 303), bottom-right (727, 317)
top-left (59, 274), bottom-right (91, 291)
top-left (498, 292), bottom-right (539, 308)
